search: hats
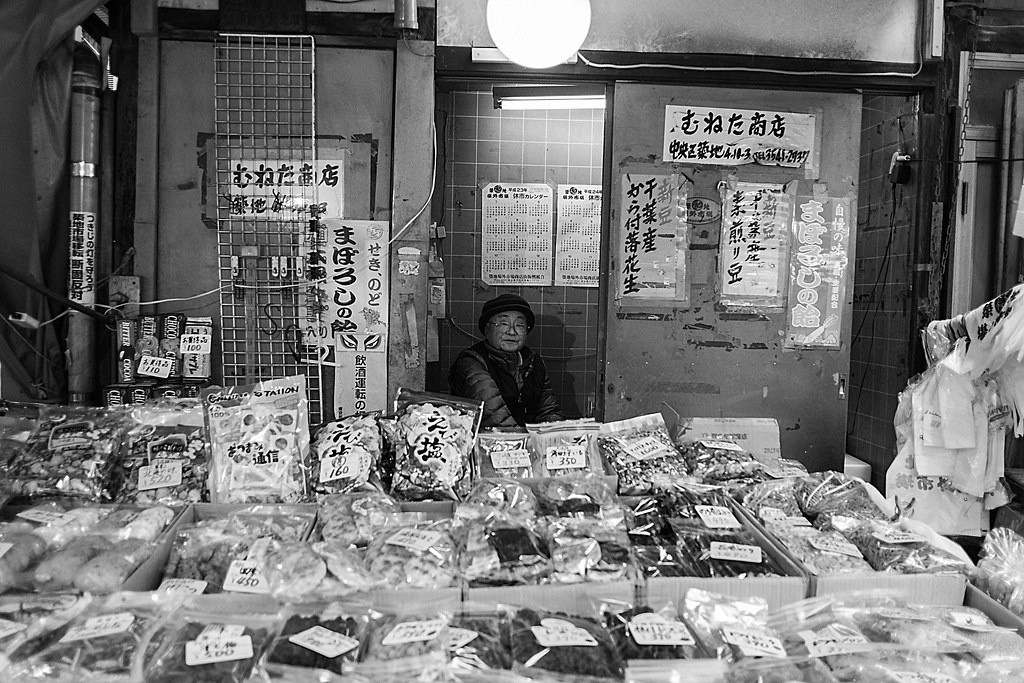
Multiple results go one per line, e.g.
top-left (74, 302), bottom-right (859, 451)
top-left (478, 293), bottom-right (535, 336)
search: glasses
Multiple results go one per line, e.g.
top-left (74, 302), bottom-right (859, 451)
top-left (490, 320), bottom-right (530, 335)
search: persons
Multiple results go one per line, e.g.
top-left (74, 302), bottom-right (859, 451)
top-left (447, 294), bottom-right (566, 428)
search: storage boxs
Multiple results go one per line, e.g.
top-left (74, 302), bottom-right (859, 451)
top-left (0, 376), bottom-right (1024, 683)
